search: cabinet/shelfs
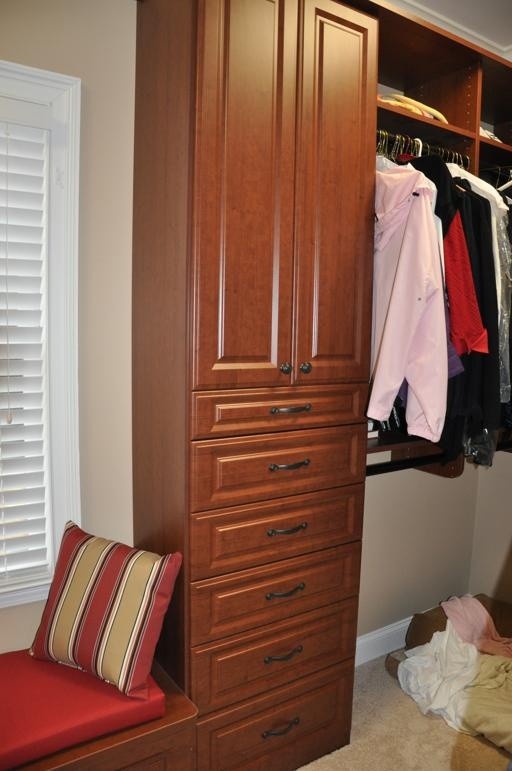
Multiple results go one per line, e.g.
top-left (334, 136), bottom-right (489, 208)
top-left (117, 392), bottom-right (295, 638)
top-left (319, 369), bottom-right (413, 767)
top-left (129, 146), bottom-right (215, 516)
top-left (131, 0), bottom-right (512, 770)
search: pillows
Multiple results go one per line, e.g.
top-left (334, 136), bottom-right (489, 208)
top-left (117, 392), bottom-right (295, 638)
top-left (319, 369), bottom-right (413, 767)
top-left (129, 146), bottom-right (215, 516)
top-left (28, 519), bottom-right (182, 700)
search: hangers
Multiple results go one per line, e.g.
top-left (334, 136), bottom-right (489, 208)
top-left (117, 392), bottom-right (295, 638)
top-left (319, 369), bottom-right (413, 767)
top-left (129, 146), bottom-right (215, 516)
top-left (373, 126), bottom-right (512, 189)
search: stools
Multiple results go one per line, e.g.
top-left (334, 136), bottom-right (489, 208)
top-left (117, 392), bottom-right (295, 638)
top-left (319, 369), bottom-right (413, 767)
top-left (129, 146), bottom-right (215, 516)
top-left (2, 650), bottom-right (199, 770)
